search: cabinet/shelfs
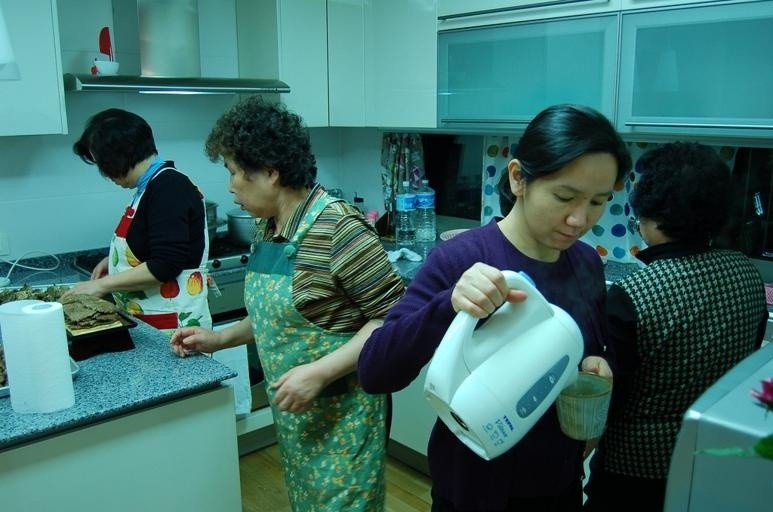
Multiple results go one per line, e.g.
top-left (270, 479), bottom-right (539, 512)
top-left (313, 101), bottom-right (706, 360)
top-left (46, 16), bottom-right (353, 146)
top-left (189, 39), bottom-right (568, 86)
top-left (621, 1), bottom-right (771, 148)
top-left (235, 1), bottom-right (328, 129)
top-left (438, 13), bottom-right (621, 142)
top-left (0, 0), bottom-right (70, 135)
top-left (328, 0), bottom-right (438, 128)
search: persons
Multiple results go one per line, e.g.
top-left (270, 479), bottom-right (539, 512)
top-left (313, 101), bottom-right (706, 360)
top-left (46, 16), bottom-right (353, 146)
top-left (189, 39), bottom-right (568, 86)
top-left (356, 106), bottom-right (634, 512)
top-left (583, 140), bottom-right (771, 512)
top-left (60, 108), bottom-right (214, 358)
top-left (170, 95), bottom-right (406, 511)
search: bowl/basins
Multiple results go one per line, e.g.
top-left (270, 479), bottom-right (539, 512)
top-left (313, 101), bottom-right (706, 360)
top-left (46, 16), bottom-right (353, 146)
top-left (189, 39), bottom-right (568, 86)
top-left (92, 60), bottom-right (120, 76)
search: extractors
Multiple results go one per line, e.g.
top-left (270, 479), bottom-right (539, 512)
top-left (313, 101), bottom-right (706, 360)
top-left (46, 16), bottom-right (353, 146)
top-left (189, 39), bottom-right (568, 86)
top-left (62, 0), bottom-right (291, 96)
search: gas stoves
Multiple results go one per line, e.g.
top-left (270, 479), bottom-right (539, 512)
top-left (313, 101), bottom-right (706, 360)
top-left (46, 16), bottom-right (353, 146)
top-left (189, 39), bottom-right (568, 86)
top-left (207, 244), bottom-right (252, 273)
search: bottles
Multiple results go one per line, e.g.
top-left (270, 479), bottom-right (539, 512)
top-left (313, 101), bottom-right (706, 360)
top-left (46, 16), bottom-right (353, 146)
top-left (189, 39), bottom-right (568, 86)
top-left (416, 179), bottom-right (437, 244)
top-left (395, 181), bottom-right (416, 249)
top-left (366, 209), bottom-right (379, 229)
top-left (353, 196), bottom-right (364, 213)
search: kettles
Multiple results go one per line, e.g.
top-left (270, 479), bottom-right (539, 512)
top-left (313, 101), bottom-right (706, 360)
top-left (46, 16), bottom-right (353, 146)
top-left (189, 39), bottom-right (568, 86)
top-left (422, 269), bottom-right (585, 462)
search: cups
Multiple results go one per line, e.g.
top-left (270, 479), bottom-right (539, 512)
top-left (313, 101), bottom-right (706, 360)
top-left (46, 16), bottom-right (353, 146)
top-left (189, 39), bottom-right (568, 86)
top-left (553, 371), bottom-right (615, 441)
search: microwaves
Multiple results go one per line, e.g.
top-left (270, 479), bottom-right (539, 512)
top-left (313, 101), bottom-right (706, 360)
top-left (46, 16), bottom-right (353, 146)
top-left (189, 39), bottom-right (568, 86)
top-left (659, 341), bottom-right (773, 512)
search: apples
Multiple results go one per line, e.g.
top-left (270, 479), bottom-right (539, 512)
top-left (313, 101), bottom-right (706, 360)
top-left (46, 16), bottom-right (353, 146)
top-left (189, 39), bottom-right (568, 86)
top-left (160, 279), bottom-right (179, 301)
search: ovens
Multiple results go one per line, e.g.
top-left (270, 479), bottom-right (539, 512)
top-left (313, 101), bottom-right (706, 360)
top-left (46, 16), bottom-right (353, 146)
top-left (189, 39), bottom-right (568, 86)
top-left (203, 279), bottom-right (271, 413)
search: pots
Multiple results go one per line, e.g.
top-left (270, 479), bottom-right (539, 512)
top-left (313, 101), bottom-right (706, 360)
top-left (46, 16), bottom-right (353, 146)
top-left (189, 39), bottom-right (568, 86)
top-left (205, 199), bottom-right (219, 244)
top-left (226, 205), bottom-right (263, 250)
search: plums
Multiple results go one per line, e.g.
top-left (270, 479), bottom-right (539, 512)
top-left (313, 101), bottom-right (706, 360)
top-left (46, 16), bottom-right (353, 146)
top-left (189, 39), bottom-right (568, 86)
top-left (186, 272), bottom-right (203, 295)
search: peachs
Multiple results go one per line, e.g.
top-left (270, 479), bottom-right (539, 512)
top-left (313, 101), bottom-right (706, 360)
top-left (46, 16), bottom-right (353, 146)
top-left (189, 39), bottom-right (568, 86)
top-left (125, 302), bottom-right (144, 314)
top-left (126, 247), bottom-right (141, 267)
